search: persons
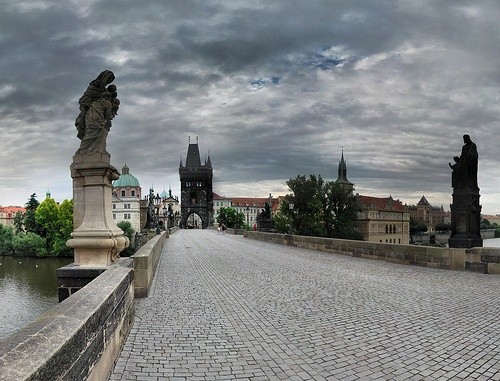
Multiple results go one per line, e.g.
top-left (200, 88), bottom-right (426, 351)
top-left (448, 134), bottom-right (479, 190)
top-left (74, 70), bottom-right (119, 156)
top-left (218, 225), bottom-right (221, 233)
top-left (222, 223), bottom-right (227, 235)
